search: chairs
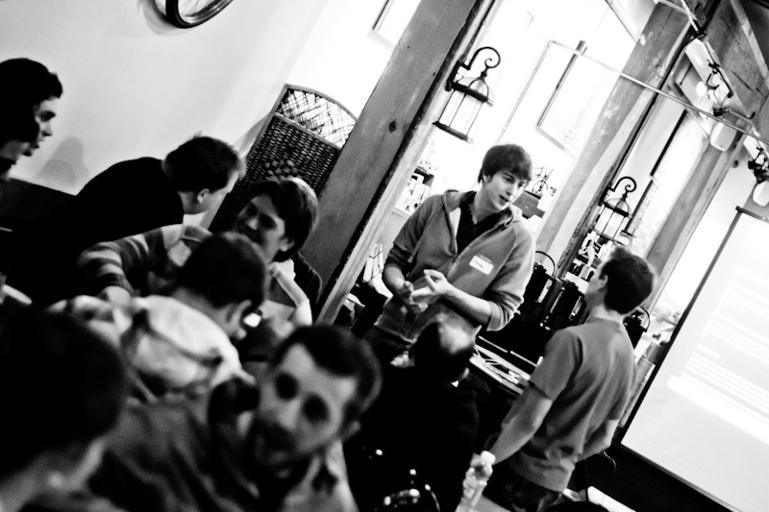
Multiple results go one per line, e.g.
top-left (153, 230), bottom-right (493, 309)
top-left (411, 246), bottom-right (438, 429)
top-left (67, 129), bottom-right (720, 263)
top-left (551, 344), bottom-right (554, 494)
top-left (0, 177), bottom-right (73, 276)
top-left (293, 251), bottom-right (321, 309)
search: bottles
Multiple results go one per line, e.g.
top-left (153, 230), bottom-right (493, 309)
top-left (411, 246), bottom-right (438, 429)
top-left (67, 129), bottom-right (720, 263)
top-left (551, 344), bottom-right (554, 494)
top-left (456, 449), bottom-right (497, 512)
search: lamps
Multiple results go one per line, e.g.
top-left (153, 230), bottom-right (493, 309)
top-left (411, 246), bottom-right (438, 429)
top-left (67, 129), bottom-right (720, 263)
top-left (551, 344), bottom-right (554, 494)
top-left (592, 176), bottom-right (636, 241)
top-left (432, 46), bottom-right (501, 141)
top-left (705, 62), bottom-right (769, 207)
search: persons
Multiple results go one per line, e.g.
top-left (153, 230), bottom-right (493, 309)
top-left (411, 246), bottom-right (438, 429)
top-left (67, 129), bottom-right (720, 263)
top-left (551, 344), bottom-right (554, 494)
top-left (468, 248), bottom-right (656, 511)
top-left (359, 143), bottom-right (538, 430)
top-left (0, 58), bottom-right (63, 159)
